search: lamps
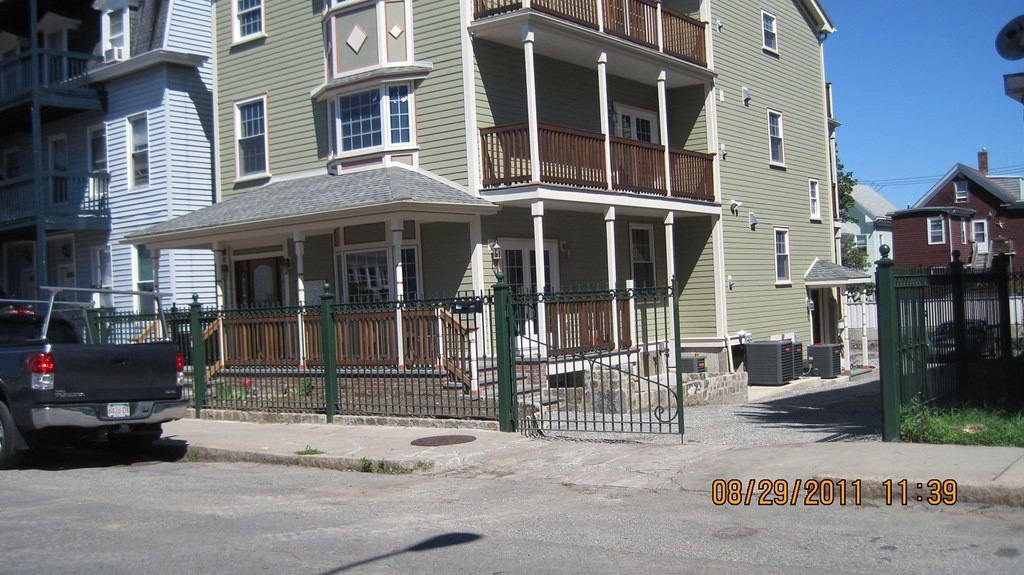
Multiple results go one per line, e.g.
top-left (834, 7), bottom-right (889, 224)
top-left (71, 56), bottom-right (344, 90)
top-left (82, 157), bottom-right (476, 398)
top-left (282, 257), bottom-right (292, 274)
top-left (488, 239), bottom-right (501, 259)
top-left (806, 298), bottom-right (814, 310)
top-left (561, 241), bottom-right (571, 259)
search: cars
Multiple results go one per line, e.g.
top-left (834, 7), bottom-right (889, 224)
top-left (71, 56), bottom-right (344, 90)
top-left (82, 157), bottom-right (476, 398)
top-left (927, 319), bottom-right (995, 363)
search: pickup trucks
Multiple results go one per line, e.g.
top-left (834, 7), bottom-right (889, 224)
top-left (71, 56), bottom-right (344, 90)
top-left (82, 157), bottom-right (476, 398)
top-left (0, 286), bottom-right (189, 470)
